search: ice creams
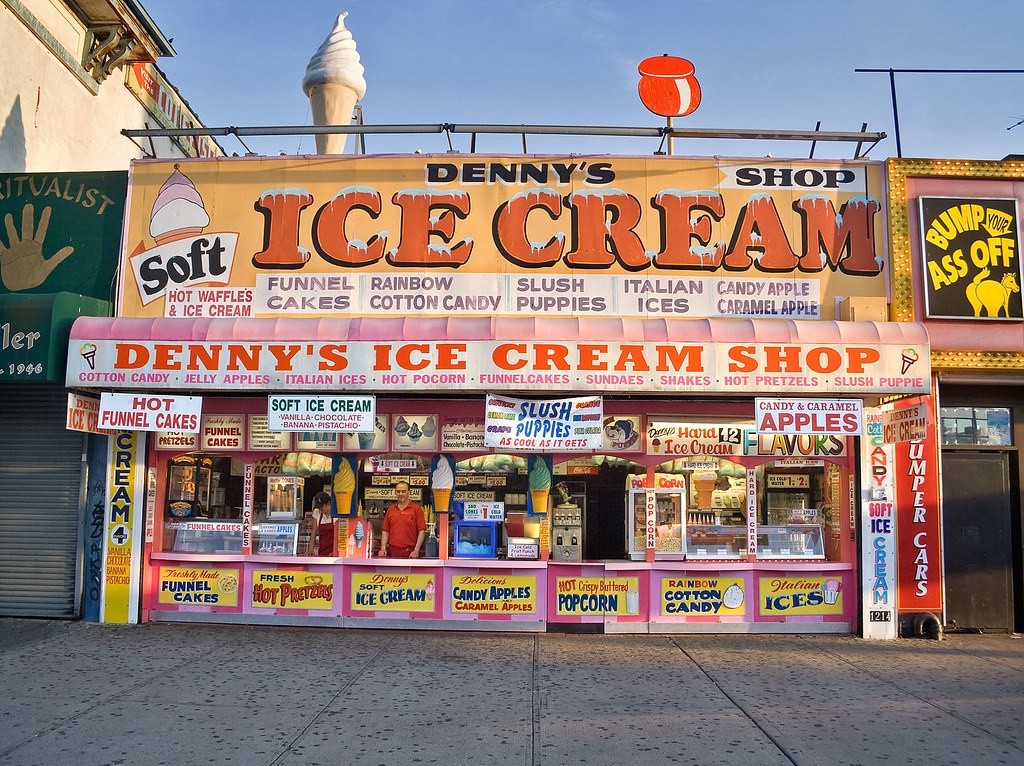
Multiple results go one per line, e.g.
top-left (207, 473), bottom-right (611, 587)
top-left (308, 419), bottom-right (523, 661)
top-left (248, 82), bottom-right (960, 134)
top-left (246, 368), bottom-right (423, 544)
top-left (431, 454), bottom-right (454, 512)
top-left (693, 470), bottom-right (717, 508)
top-left (302, 11), bottom-right (367, 155)
top-left (334, 457), bottom-right (355, 514)
top-left (355, 521), bottom-right (364, 548)
top-left (348, 533), bottom-right (355, 555)
top-left (528, 455), bottom-right (550, 513)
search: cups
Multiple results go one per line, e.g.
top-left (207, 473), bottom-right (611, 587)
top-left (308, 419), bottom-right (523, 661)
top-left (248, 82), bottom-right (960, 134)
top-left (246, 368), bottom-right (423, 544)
top-left (626, 592), bottom-right (638, 613)
top-left (512, 494), bottom-right (518, 504)
top-left (519, 494), bottom-right (525, 504)
top-left (358, 433), bottom-right (376, 449)
top-left (504, 494), bottom-right (511, 504)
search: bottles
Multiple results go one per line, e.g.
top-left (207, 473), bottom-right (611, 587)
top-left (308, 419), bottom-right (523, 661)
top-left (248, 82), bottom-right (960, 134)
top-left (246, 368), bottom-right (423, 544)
top-left (555, 509), bottom-right (559, 520)
top-left (571, 529), bottom-right (577, 545)
top-left (479, 537), bottom-right (488, 547)
top-left (567, 509), bottom-right (572, 520)
top-left (560, 509), bottom-right (565, 520)
top-left (575, 509), bottom-right (581, 520)
top-left (556, 531), bottom-right (562, 545)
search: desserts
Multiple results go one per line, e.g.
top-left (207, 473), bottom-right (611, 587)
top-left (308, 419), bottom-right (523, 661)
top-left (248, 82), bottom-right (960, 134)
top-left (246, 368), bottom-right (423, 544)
top-left (395, 416), bottom-right (409, 436)
top-left (407, 422), bottom-right (422, 441)
top-left (422, 415), bottom-right (436, 437)
